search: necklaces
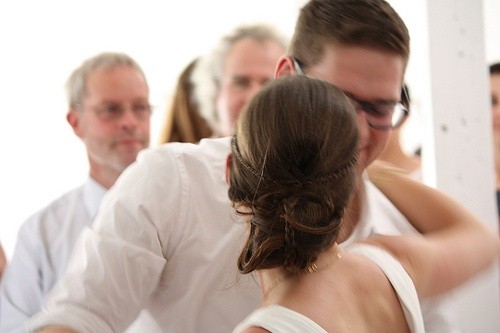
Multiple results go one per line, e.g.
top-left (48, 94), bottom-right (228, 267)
top-left (260, 246), bottom-right (343, 297)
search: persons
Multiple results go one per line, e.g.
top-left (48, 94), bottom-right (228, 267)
top-left (225, 75), bottom-right (500, 333)
top-left (0, 26), bottom-right (500, 277)
top-left (23, 0), bottom-right (459, 333)
top-left (0, 53), bottom-right (152, 333)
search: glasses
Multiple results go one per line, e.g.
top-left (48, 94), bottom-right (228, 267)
top-left (78, 99), bottom-right (155, 122)
top-left (289, 55), bottom-right (410, 131)
